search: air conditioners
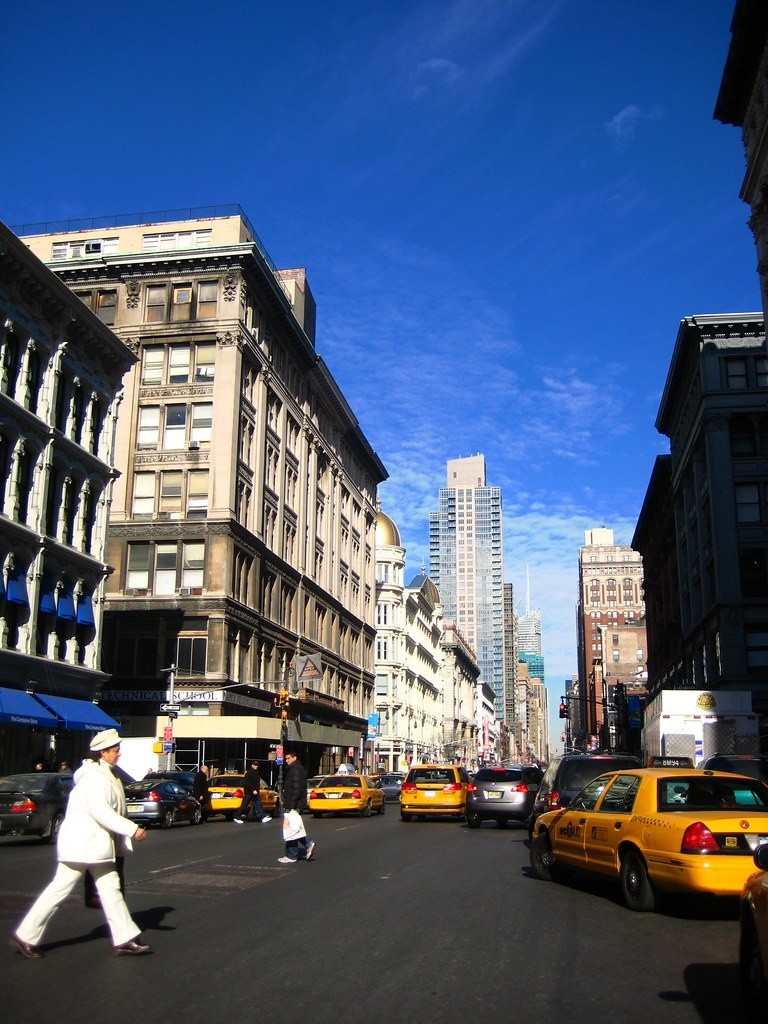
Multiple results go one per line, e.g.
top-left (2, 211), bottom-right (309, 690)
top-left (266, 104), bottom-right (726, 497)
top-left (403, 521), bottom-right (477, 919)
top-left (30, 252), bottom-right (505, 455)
top-left (188, 440), bottom-right (200, 450)
top-left (179, 588), bottom-right (190, 595)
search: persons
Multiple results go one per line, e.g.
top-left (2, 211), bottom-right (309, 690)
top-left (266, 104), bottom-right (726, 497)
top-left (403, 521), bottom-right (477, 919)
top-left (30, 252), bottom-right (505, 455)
top-left (192, 765), bottom-right (209, 824)
top-left (146, 767), bottom-right (153, 775)
top-left (58, 762), bottom-right (72, 775)
top-left (278, 752), bottom-right (315, 863)
top-left (33, 762), bottom-right (46, 773)
top-left (8, 729), bottom-right (152, 958)
top-left (233, 762), bottom-right (272, 824)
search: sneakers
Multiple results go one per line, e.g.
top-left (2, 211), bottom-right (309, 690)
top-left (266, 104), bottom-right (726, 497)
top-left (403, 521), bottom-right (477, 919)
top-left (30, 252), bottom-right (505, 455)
top-left (305, 841), bottom-right (316, 859)
top-left (278, 856), bottom-right (296, 863)
top-left (261, 816), bottom-right (272, 823)
top-left (234, 818), bottom-right (243, 824)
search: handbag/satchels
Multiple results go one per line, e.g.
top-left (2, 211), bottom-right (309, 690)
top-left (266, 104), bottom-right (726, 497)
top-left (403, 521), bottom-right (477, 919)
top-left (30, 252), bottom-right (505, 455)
top-left (83, 856), bottom-right (125, 908)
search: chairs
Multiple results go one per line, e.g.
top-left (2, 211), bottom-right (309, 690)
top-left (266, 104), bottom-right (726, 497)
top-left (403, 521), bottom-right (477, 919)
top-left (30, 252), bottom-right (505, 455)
top-left (415, 776), bottom-right (450, 783)
top-left (686, 789), bottom-right (714, 807)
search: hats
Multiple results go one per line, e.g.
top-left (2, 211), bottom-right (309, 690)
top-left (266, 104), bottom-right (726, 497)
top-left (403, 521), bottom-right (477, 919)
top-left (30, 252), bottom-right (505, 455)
top-left (89, 728), bottom-right (123, 751)
top-left (252, 760), bottom-right (260, 765)
top-left (61, 761), bottom-right (66, 765)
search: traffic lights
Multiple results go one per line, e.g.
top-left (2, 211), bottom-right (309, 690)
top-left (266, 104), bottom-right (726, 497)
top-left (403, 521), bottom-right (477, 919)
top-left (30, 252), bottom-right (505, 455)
top-left (559, 704), bottom-right (566, 719)
top-left (160, 703), bottom-right (180, 712)
top-left (280, 691), bottom-right (289, 708)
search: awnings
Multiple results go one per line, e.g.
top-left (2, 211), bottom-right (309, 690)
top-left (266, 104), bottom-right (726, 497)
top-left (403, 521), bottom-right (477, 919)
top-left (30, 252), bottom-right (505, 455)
top-left (0, 687), bottom-right (124, 733)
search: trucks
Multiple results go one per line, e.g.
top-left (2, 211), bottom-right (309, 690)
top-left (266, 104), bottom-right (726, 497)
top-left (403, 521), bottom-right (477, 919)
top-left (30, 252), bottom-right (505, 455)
top-left (641, 689), bottom-right (760, 760)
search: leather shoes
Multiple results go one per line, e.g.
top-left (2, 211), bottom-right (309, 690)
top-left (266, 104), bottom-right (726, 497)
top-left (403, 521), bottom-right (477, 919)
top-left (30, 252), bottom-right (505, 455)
top-left (12, 935), bottom-right (42, 958)
top-left (114, 938), bottom-right (152, 956)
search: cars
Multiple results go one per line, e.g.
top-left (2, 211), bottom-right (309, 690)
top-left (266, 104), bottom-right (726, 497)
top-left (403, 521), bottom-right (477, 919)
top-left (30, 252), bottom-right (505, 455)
top-left (123, 778), bottom-right (202, 828)
top-left (401, 764), bottom-right (474, 822)
top-left (0, 772), bottom-right (73, 845)
top-left (206, 770), bottom-right (282, 820)
top-left (144, 772), bottom-right (196, 796)
top-left (531, 756), bottom-right (768, 913)
top-left (309, 763), bottom-right (386, 818)
top-left (307, 774), bottom-right (327, 805)
top-left (370, 775), bottom-right (405, 802)
top-left (737, 843), bottom-right (768, 1024)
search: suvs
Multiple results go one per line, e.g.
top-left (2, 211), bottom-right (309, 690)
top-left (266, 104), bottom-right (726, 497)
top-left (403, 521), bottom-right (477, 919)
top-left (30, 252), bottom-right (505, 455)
top-left (466, 764), bottom-right (547, 829)
top-left (696, 754), bottom-right (768, 806)
top-left (528, 749), bottom-right (644, 839)
